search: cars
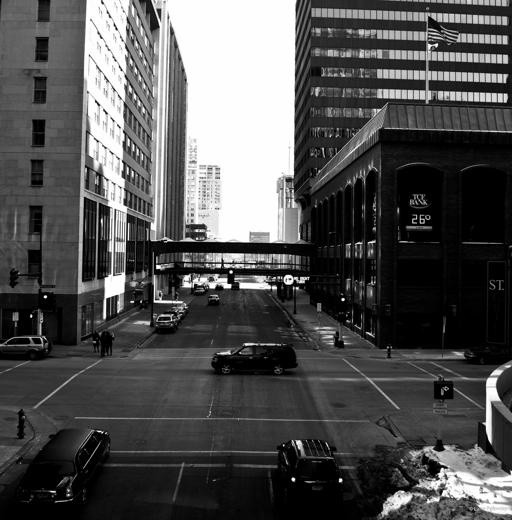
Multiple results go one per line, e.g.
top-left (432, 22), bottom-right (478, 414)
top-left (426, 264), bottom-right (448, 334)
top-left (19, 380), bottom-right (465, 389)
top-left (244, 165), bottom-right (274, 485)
top-left (194, 273), bottom-right (224, 296)
top-left (14, 427), bottom-right (113, 512)
top-left (463, 342), bottom-right (511, 364)
top-left (232, 280), bottom-right (240, 291)
top-left (155, 300), bottom-right (189, 332)
top-left (208, 295), bottom-right (221, 304)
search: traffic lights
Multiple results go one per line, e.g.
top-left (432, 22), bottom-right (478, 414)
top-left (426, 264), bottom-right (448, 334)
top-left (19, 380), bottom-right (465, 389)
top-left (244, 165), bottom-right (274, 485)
top-left (8, 267), bottom-right (20, 289)
top-left (42, 291), bottom-right (54, 301)
top-left (227, 268), bottom-right (235, 284)
top-left (339, 293), bottom-right (347, 311)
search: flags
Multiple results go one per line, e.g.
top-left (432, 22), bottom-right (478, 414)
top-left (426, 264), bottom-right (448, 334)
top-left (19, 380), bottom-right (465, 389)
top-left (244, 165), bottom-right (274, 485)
top-left (426, 15), bottom-right (460, 48)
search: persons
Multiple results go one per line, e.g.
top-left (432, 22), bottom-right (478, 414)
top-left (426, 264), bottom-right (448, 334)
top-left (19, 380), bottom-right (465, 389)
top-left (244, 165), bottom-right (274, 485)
top-left (91, 328), bottom-right (116, 359)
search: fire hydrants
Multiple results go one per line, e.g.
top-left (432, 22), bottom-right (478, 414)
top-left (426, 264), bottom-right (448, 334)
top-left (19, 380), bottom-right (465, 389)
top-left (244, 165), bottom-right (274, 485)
top-left (385, 344), bottom-right (394, 359)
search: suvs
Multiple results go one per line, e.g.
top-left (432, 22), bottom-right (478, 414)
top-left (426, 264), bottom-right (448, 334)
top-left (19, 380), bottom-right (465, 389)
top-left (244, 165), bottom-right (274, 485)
top-left (211, 341), bottom-right (300, 374)
top-left (0, 336), bottom-right (52, 359)
top-left (274, 437), bottom-right (347, 511)
